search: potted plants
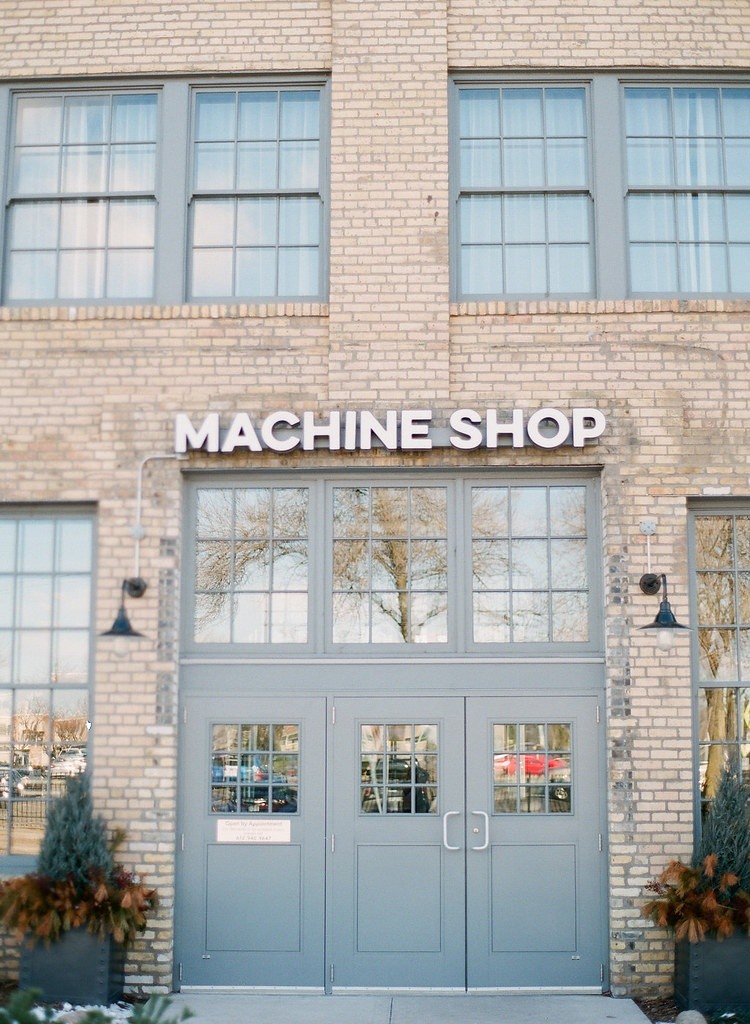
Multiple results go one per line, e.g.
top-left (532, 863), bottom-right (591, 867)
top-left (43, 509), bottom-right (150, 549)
top-left (648, 758), bottom-right (750, 1016)
top-left (21, 771), bottom-right (128, 1003)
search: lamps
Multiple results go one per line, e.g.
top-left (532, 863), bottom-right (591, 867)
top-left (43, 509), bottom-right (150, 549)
top-left (96, 578), bottom-right (150, 657)
top-left (637, 571), bottom-right (696, 651)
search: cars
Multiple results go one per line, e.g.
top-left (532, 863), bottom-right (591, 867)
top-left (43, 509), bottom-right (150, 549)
top-left (494, 745), bottom-right (570, 802)
top-left (361, 760), bottom-right (433, 813)
top-left (211, 755), bottom-right (297, 814)
top-left (0, 766), bottom-right (48, 802)
top-left (49, 748), bottom-right (86, 778)
